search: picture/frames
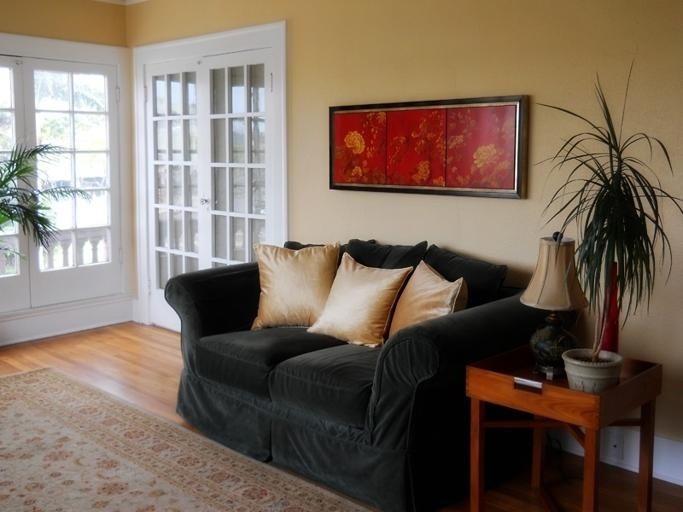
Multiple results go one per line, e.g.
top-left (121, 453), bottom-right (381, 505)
top-left (326, 93), bottom-right (532, 202)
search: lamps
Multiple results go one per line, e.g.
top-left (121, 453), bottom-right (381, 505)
top-left (518, 227), bottom-right (590, 366)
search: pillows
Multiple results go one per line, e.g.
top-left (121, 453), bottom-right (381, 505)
top-left (388, 256), bottom-right (469, 340)
top-left (307, 251), bottom-right (413, 350)
top-left (246, 237), bottom-right (336, 334)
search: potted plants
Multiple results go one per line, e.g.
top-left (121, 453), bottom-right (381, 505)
top-left (530, 54), bottom-right (682, 393)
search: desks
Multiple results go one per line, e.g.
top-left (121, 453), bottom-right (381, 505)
top-left (462, 352), bottom-right (662, 511)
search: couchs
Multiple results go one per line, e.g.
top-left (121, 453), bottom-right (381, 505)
top-left (158, 224), bottom-right (544, 510)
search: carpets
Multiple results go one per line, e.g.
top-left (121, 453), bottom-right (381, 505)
top-left (0, 359), bottom-right (372, 511)
